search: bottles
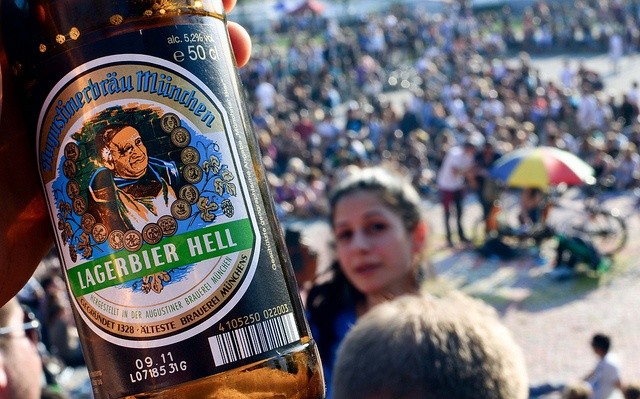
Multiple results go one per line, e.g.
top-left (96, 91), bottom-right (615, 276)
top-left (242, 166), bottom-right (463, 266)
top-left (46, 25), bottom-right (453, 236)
top-left (0, 0), bottom-right (326, 399)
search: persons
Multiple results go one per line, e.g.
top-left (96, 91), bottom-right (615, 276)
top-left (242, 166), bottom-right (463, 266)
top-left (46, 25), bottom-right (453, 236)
top-left (85, 122), bottom-right (182, 231)
top-left (579, 331), bottom-right (626, 398)
top-left (331, 287), bottom-right (529, 399)
top-left (0, 292), bottom-right (43, 399)
top-left (303, 165), bottom-right (427, 394)
top-left (1, 1), bottom-right (251, 304)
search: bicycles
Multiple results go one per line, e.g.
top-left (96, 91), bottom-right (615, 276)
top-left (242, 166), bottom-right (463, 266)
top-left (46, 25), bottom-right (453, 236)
top-left (573, 206), bottom-right (627, 256)
